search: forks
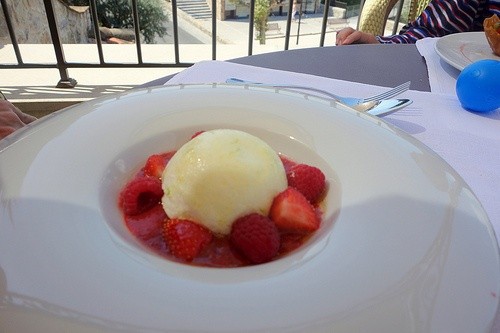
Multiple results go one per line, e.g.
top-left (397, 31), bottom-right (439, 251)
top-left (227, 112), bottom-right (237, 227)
top-left (226, 77), bottom-right (411, 105)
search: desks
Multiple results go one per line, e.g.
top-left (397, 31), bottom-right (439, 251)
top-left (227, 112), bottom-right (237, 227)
top-left (129, 44), bottom-right (500, 333)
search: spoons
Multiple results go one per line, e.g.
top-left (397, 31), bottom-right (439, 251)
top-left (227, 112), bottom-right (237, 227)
top-left (365, 99), bottom-right (412, 117)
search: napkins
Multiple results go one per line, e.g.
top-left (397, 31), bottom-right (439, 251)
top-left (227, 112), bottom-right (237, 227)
top-left (414, 35), bottom-right (462, 96)
top-left (164, 60), bottom-right (499, 263)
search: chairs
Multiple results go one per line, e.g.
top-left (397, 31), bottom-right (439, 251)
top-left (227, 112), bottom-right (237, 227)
top-left (358, 0), bottom-right (432, 37)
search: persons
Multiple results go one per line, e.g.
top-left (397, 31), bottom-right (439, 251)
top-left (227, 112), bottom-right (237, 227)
top-left (336, 0), bottom-right (500, 44)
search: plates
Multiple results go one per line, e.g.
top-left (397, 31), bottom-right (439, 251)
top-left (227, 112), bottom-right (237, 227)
top-left (434, 32), bottom-right (500, 71)
top-left (165, 60), bottom-right (500, 249)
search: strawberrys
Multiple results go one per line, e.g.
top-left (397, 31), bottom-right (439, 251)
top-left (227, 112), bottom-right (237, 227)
top-left (122, 130), bottom-right (326, 266)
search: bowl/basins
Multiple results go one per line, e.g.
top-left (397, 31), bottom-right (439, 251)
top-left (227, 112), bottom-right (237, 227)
top-left (0, 85), bottom-right (500, 333)
